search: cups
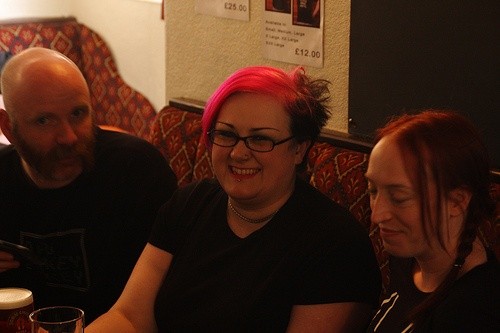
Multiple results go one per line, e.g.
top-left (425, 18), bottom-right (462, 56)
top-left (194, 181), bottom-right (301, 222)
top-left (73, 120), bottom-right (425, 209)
top-left (0, 288), bottom-right (34, 333)
top-left (29, 306), bottom-right (85, 333)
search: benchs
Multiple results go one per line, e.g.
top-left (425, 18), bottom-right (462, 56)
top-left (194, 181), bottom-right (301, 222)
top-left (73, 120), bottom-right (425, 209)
top-left (148, 96), bottom-right (500, 302)
top-left (0, 16), bottom-right (156, 138)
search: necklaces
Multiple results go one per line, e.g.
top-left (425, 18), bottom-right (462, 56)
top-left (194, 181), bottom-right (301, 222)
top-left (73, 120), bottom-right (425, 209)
top-left (228, 200), bottom-right (278, 223)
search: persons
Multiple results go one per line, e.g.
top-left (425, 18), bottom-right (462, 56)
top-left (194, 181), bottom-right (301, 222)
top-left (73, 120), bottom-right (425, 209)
top-left (365, 110), bottom-right (500, 333)
top-left (84, 66), bottom-right (382, 333)
top-left (0, 46), bottom-right (178, 333)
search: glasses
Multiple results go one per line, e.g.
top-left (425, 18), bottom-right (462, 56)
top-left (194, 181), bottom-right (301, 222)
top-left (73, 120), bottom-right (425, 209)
top-left (206, 128), bottom-right (291, 153)
